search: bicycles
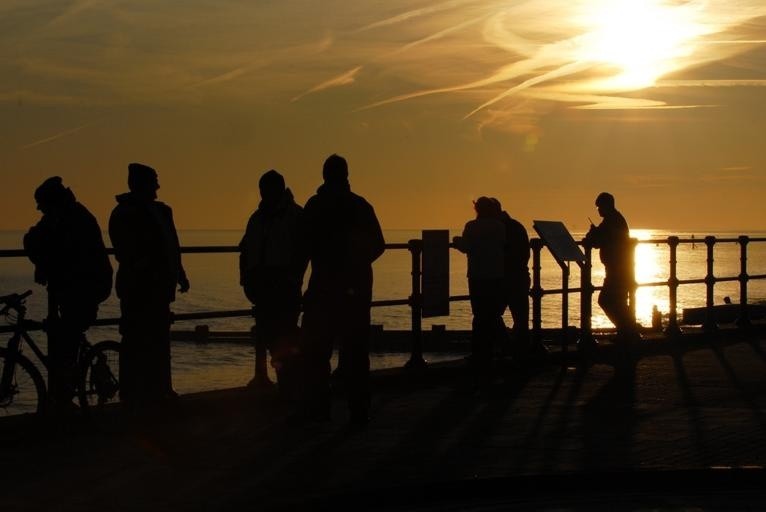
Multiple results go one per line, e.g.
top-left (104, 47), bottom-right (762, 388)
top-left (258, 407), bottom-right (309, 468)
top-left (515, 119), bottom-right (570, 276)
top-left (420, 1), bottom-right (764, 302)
top-left (0, 287), bottom-right (123, 422)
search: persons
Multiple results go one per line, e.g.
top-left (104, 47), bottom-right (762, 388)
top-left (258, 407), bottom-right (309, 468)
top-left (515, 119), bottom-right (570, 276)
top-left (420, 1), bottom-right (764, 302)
top-left (452, 195), bottom-right (513, 340)
top-left (237, 170), bottom-right (320, 387)
top-left (488, 198), bottom-right (532, 334)
top-left (107, 161), bottom-right (192, 404)
top-left (579, 191), bottom-right (648, 343)
top-left (24, 174), bottom-right (114, 414)
top-left (296, 152), bottom-right (386, 381)
